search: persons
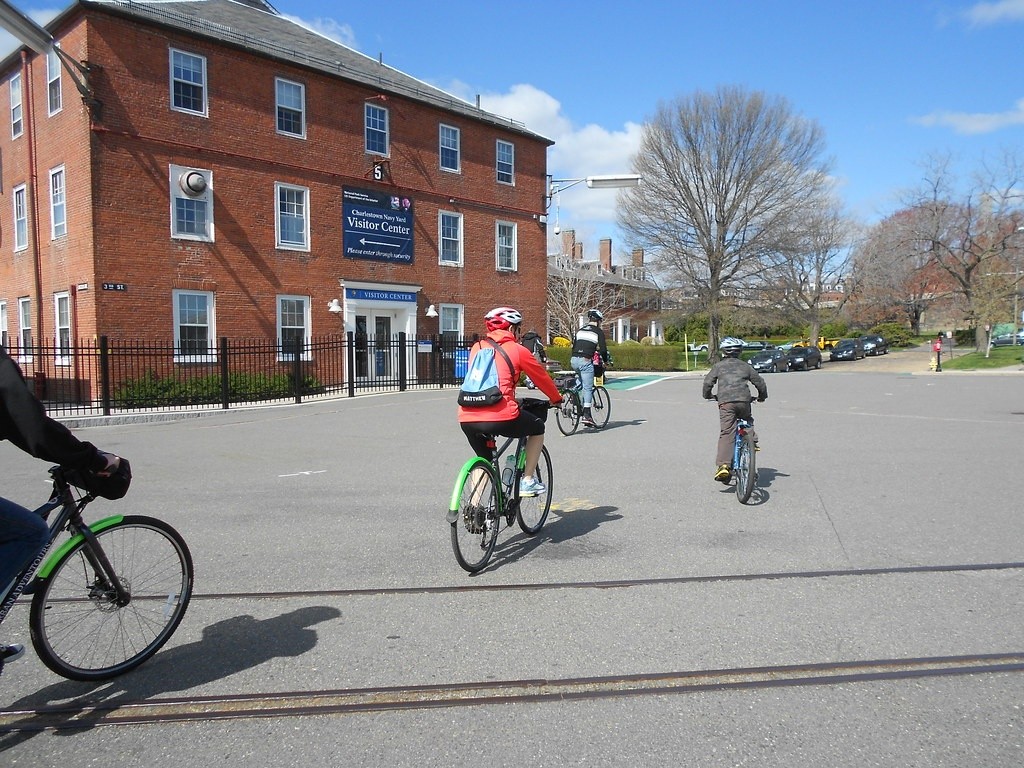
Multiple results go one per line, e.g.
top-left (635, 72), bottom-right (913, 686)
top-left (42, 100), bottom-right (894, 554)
top-left (522, 326), bottom-right (547, 389)
top-left (459, 307), bottom-right (562, 533)
top-left (571, 309), bottom-right (608, 426)
top-left (703, 337), bottom-right (768, 480)
top-left (0, 345), bottom-right (109, 664)
top-left (938, 330), bottom-right (943, 337)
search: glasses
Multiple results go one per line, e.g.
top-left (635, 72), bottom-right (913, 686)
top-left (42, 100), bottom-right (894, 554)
top-left (508, 323), bottom-right (521, 334)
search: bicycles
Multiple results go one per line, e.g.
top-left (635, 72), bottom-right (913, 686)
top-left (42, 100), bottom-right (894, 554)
top-left (445, 398), bottom-right (567, 574)
top-left (1, 463), bottom-right (194, 683)
top-left (705, 394), bottom-right (766, 504)
top-left (554, 357), bottom-right (614, 436)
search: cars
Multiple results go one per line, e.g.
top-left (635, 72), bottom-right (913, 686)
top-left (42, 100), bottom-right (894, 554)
top-left (859, 334), bottom-right (889, 356)
top-left (784, 346), bottom-right (822, 371)
top-left (774, 339), bottom-right (804, 350)
top-left (747, 349), bottom-right (789, 373)
top-left (830, 338), bottom-right (866, 362)
top-left (989, 327), bottom-right (1024, 348)
top-left (687, 336), bottom-right (775, 351)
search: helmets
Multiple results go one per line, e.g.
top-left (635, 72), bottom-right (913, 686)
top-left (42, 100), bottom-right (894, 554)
top-left (719, 337), bottom-right (742, 355)
top-left (484, 306), bottom-right (522, 332)
top-left (588, 309), bottom-right (603, 319)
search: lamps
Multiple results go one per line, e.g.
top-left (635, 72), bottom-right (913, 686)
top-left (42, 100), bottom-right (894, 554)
top-left (541, 171), bottom-right (645, 201)
top-left (363, 94), bottom-right (390, 102)
top-left (0, 0), bottom-right (105, 124)
top-left (326, 298), bottom-right (344, 315)
top-left (425, 304), bottom-right (439, 319)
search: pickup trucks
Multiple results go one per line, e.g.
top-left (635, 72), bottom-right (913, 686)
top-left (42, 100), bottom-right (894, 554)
top-left (793, 337), bottom-right (840, 352)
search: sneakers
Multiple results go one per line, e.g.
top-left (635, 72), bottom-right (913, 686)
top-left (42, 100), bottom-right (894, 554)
top-left (754, 442), bottom-right (761, 451)
top-left (581, 413), bottom-right (596, 427)
top-left (0, 643), bottom-right (26, 663)
top-left (573, 405), bottom-right (584, 416)
top-left (519, 473), bottom-right (547, 497)
top-left (714, 464), bottom-right (730, 481)
top-left (464, 506), bottom-right (482, 534)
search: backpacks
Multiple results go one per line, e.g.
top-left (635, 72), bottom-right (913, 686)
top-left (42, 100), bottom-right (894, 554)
top-left (457, 340), bottom-right (511, 407)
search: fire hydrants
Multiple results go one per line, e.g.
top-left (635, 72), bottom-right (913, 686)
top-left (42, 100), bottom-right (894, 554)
top-left (928, 357), bottom-right (938, 371)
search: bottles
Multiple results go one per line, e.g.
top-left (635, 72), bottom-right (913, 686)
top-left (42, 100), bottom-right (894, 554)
top-left (503, 455), bottom-right (518, 483)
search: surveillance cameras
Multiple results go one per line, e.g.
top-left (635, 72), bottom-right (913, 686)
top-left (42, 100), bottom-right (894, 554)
top-left (554, 227), bottom-right (561, 235)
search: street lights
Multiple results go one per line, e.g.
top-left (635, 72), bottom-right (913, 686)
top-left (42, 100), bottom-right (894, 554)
top-left (985, 267), bottom-right (1023, 346)
